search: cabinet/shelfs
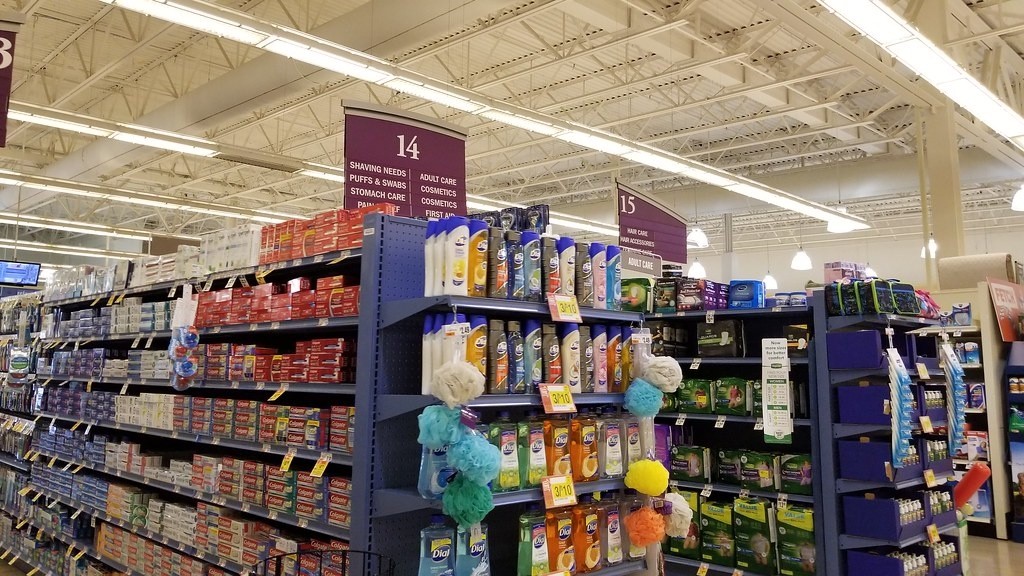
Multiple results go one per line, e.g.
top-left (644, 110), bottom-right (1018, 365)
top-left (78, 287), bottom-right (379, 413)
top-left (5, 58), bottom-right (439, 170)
top-left (645, 291), bottom-right (965, 576)
top-left (928, 282), bottom-right (1024, 539)
top-left (0, 213), bottom-right (672, 576)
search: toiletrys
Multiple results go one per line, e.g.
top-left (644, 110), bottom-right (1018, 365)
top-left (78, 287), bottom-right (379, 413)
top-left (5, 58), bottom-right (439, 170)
top-left (901, 445), bottom-right (919, 467)
top-left (421, 312), bottom-right (636, 396)
top-left (910, 391), bottom-right (917, 411)
top-left (924, 390), bottom-right (944, 409)
top-left (890, 498), bottom-right (925, 526)
top-left (10, 200), bottom-right (400, 576)
top-left (416, 405), bottom-right (643, 500)
top-left (516, 488), bottom-right (647, 576)
top-left (925, 439), bottom-right (951, 462)
top-left (423, 216), bottom-right (622, 312)
top-left (886, 550), bottom-right (929, 576)
top-left (417, 514), bottom-right (492, 576)
top-left (918, 539), bottom-right (958, 571)
top-left (918, 490), bottom-right (954, 516)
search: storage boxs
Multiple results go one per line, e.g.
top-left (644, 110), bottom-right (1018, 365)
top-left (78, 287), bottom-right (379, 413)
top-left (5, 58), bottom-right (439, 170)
top-left (316, 289), bottom-right (343, 304)
top-left (329, 553), bottom-right (350, 567)
top-left (677, 279), bottom-right (718, 311)
top-left (0, 220), bottom-right (329, 576)
top-left (315, 210), bottom-right (349, 225)
top-left (312, 339), bottom-right (352, 353)
top-left (328, 476), bottom-right (352, 495)
top-left (323, 567), bottom-right (349, 576)
top-left (337, 233), bottom-right (363, 250)
top-left (343, 286), bottom-right (361, 317)
top-left (313, 237), bottom-right (337, 255)
top-left (315, 223), bottom-right (338, 242)
top-left (338, 203), bottom-right (395, 236)
top-left (328, 507), bottom-right (350, 528)
top-left (328, 492), bottom-right (351, 510)
top-left (317, 275), bottom-right (344, 290)
top-left (310, 354), bottom-right (350, 369)
top-left (316, 304), bottom-right (343, 318)
top-left (329, 406), bottom-right (355, 453)
top-left (329, 540), bottom-right (349, 556)
top-left (308, 369), bottom-right (350, 384)
top-left (718, 282), bottom-right (730, 310)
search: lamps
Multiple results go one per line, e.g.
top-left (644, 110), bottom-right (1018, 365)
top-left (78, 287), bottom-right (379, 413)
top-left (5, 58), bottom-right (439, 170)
top-left (790, 213), bottom-right (813, 270)
top-left (827, 130), bottom-right (857, 233)
top-left (921, 200), bottom-right (937, 258)
top-left (688, 249), bottom-right (706, 279)
top-left (686, 180), bottom-right (709, 248)
top-left (762, 239), bottom-right (778, 290)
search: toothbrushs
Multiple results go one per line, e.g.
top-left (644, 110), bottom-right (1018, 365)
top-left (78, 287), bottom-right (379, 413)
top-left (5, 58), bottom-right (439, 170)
top-left (0, 381), bottom-right (43, 415)
top-left (0, 304), bottom-right (38, 333)
top-left (0, 467), bottom-right (44, 546)
top-left (25, 342), bottom-right (54, 374)
top-left (0, 428), bottom-right (32, 461)
top-left (0, 342), bottom-right (13, 371)
